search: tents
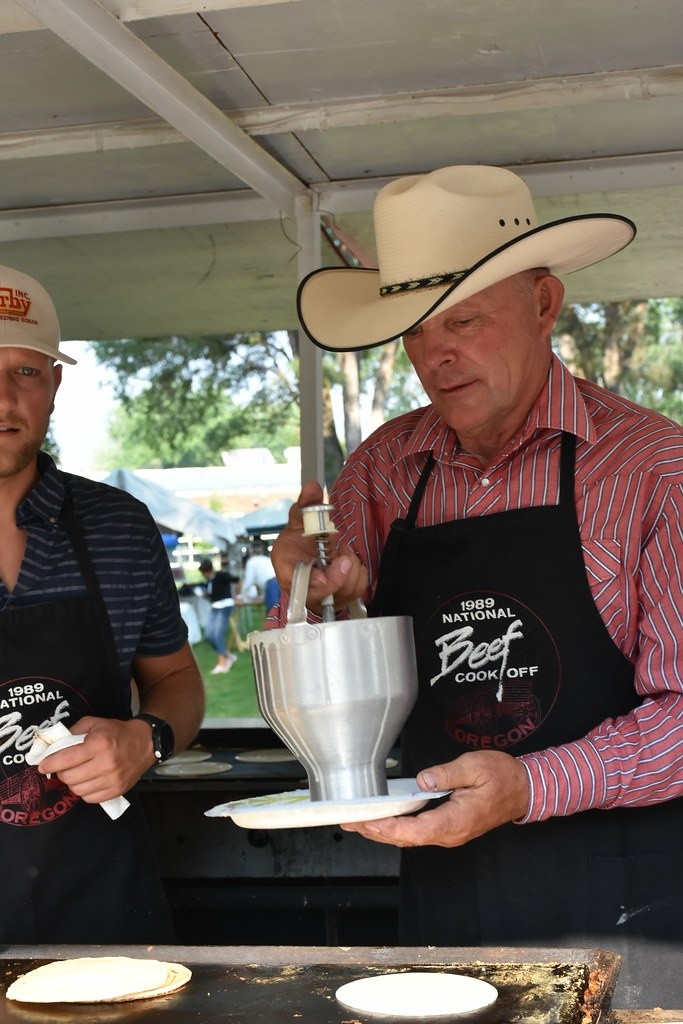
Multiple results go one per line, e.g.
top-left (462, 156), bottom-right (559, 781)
top-left (102, 466), bottom-right (302, 563)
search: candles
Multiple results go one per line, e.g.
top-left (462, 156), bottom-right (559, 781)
top-left (302, 482), bottom-right (337, 536)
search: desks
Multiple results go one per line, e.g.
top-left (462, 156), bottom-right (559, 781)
top-left (225, 598), bottom-right (265, 651)
top-left (180, 600), bottom-right (202, 644)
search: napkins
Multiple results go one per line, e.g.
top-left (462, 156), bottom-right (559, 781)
top-left (25, 721), bottom-right (131, 822)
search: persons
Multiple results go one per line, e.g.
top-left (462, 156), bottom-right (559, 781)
top-left (271, 165), bottom-right (683, 1009)
top-left (0, 265), bottom-right (205, 952)
top-left (199, 540), bottom-right (281, 674)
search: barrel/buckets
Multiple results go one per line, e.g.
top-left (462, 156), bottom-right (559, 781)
top-left (249, 558), bottom-right (417, 803)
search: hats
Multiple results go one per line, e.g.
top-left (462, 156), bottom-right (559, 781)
top-left (295, 163), bottom-right (637, 352)
top-left (0, 265), bottom-right (77, 366)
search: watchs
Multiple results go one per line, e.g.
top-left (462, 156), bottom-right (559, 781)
top-left (132, 713), bottom-right (175, 764)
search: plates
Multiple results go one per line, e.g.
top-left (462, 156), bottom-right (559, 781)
top-left (203, 777), bottom-right (455, 830)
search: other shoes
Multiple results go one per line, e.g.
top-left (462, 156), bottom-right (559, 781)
top-left (211, 654), bottom-right (236, 673)
